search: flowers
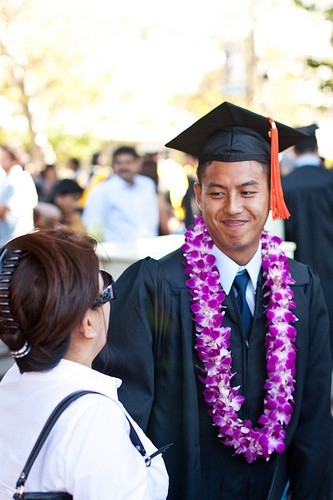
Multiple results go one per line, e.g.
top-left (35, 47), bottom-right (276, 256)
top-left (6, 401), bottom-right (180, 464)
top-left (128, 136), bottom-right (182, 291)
top-left (181, 214), bottom-right (298, 464)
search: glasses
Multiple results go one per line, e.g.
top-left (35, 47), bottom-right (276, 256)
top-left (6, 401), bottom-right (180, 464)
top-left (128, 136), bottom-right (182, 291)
top-left (91, 270), bottom-right (118, 310)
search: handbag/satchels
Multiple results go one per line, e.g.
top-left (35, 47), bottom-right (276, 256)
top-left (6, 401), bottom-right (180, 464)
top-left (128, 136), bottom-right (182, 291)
top-left (13, 391), bottom-right (174, 500)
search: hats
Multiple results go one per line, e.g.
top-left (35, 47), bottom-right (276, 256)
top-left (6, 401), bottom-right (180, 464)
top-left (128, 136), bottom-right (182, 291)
top-left (294, 124), bottom-right (319, 142)
top-left (165, 101), bottom-right (311, 220)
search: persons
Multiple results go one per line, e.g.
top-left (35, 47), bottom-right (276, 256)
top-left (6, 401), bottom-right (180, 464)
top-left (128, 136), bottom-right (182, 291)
top-left (93, 100), bottom-right (333, 499)
top-left (276, 124), bottom-right (332, 310)
top-left (0, 218), bottom-right (170, 500)
top-left (1, 124), bottom-right (197, 245)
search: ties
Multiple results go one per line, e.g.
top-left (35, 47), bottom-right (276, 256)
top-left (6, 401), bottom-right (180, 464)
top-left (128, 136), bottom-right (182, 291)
top-left (232, 270), bottom-right (254, 343)
